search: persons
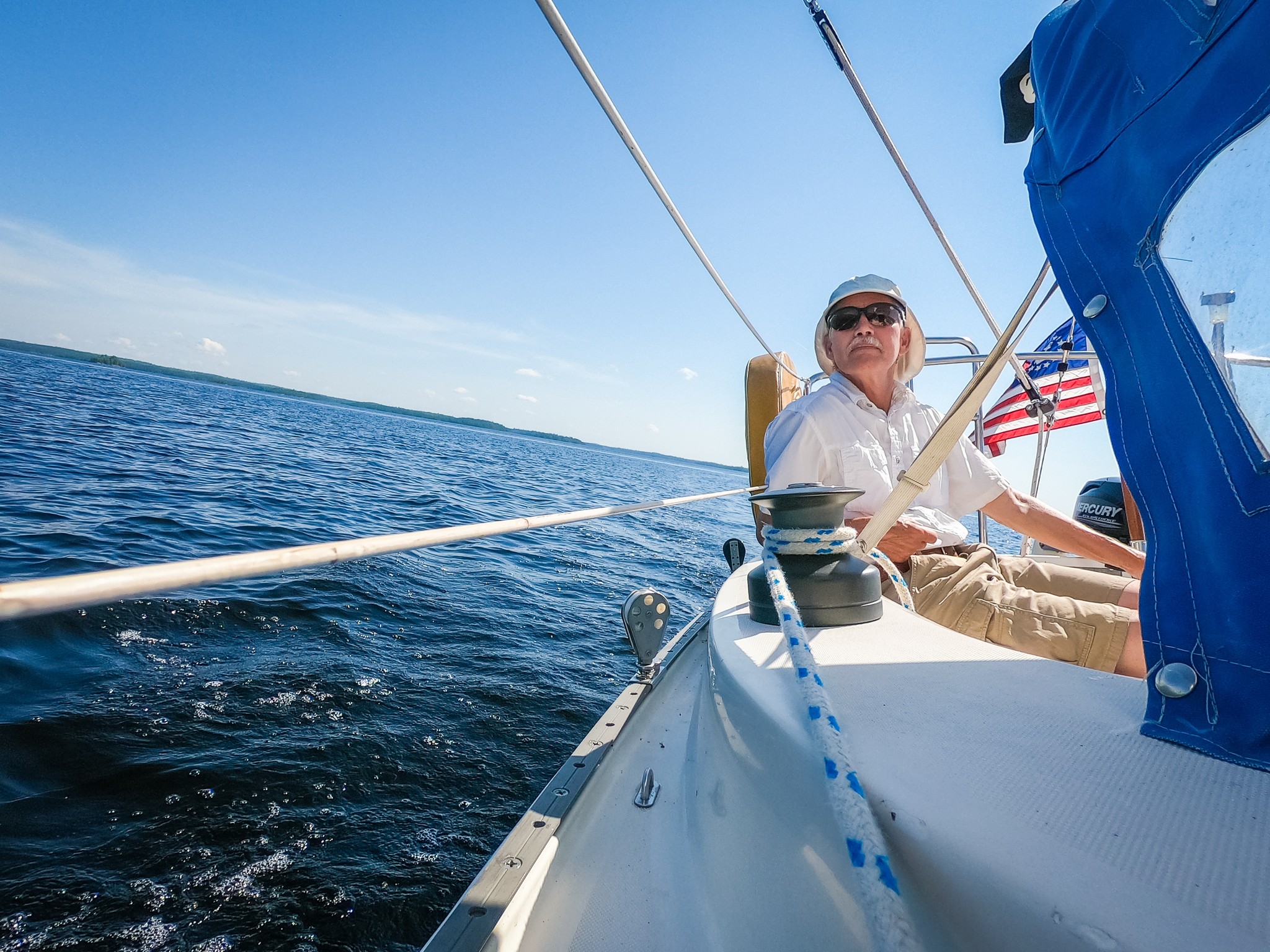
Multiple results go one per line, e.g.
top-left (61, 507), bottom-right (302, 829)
top-left (761, 275), bottom-right (1148, 681)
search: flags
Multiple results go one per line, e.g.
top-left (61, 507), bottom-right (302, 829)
top-left (969, 317), bottom-right (1102, 459)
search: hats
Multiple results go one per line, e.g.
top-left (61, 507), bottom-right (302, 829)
top-left (813, 274), bottom-right (926, 384)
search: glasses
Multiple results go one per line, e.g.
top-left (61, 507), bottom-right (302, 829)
top-left (828, 302), bottom-right (906, 332)
